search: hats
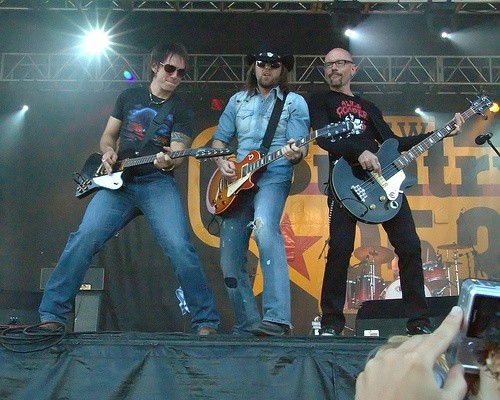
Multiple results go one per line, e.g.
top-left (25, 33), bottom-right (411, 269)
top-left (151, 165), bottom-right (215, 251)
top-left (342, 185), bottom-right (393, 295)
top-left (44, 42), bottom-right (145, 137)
top-left (245, 47), bottom-right (295, 72)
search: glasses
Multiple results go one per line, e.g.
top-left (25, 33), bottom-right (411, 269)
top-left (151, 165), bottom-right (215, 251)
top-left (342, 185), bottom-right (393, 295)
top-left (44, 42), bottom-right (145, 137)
top-left (159, 62), bottom-right (186, 77)
top-left (325, 60), bottom-right (354, 68)
top-left (256, 61), bottom-right (281, 68)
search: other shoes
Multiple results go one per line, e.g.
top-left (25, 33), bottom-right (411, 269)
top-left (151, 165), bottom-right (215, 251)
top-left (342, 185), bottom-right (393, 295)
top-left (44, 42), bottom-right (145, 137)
top-left (253, 321), bottom-right (287, 336)
top-left (197, 327), bottom-right (216, 336)
top-left (38, 322), bottom-right (62, 329)
top-left (407, 325), bottom-right (432, 336)
top-left (321, 329), bottom-right (335, 336)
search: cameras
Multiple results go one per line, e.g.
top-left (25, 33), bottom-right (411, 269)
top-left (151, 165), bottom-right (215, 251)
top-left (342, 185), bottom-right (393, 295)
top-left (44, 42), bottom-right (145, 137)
top-left (443, 278), bottom-right (500, 375)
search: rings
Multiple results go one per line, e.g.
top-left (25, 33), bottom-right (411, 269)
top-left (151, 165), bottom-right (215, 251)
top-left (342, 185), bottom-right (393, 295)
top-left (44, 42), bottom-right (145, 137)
top-left (377, 162), bottom-right (380, 166)
top-left (103, 160), bottom-right (105, 162)
top-left (287, 154), bottom-right (289, 156)
top-left (368, 166), bottom-right (371, 169)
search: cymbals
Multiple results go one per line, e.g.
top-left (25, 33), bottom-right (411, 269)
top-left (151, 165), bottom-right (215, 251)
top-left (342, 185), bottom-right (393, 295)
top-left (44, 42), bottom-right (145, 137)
top-left (442, 261), bottom-right (462, 266)
top-left (438, 243), bottom-right (471, 250)
top-left (354, 245), bottom-right (395, 265)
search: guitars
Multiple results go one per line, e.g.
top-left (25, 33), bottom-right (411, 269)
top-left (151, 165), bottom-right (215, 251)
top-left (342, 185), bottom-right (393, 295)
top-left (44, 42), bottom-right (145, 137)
top-left (73, 145), bottom-right (235, 200)
top-left (206, 119), bottom-right (354, 216)
top-left (331, 94), bottom-right (491, 225)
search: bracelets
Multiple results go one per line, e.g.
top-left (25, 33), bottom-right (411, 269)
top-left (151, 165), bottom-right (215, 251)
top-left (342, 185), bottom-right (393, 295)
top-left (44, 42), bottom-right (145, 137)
top-left (162, 163), bottom-right (175, 172)
top-left (293, 153), bottom-right (303, 164)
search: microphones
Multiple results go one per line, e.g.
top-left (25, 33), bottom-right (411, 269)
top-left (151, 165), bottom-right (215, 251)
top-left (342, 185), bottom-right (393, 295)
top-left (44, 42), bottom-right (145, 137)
top-left (475, 132), bottom-right (493, 145)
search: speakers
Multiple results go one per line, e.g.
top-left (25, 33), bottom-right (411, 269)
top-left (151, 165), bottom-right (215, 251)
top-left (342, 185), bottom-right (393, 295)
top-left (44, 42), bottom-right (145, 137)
top-left (74, 289), bottom-right (106, 334)
top-left (354, 299), bottom-right (409, 338)
top-left (425, 295), bottom-right (459, 333)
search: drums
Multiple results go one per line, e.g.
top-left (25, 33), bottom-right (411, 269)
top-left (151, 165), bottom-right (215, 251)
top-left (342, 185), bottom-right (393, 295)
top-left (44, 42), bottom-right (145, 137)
top-left (350, 274), bottom-right (384, 308)
top-left (377, 278), bottom-right (433, 301)
top-left (422, 261), bottom-right (447, 291)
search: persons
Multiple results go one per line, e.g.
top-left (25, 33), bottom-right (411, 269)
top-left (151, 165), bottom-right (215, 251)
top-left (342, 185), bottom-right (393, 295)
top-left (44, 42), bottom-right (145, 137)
top-left (212, 39), bottom-right (310, 336)
top-left (37, 42), bottom-right (221, 335)
top-left (307, 48), bottom-right (465, 337)
top-left (354, 306), bottom-right (500, 400)
